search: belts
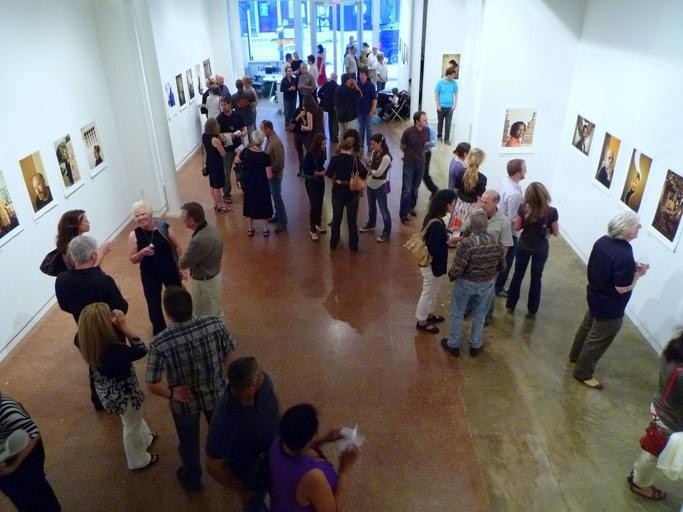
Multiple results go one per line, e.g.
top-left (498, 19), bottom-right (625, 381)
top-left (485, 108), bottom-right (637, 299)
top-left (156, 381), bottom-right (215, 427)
top-left (191, 271), bottom-right (220, 280)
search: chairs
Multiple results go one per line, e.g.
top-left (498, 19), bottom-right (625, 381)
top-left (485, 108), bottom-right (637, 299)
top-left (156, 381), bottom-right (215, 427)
top-left (384, 91), bottom-right (407, 122)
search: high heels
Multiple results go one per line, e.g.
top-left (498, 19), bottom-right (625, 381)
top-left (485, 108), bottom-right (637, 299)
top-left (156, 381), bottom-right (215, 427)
top-left (315, 223), bottom-right (327, 234)
top-left (309, 229), bottom-right (320, 241)
top-left (213, 203), bottom-right (231, 213)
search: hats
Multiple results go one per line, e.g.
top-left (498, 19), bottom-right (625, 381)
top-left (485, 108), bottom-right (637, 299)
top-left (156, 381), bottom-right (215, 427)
top-left (0, 428), bottom-right (30, 464)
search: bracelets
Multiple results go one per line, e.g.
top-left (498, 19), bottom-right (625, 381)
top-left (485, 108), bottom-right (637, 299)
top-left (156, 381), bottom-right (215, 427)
top-left (168, 387), bottom-right (173, 399)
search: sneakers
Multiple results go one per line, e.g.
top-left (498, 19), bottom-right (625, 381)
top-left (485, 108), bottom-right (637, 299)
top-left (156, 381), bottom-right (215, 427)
top-left (358, 223), bottom-right (377, 232)
top-left (416, 319), bottom-right (440, 334)
top-left (469, 343), bottom-right (484, 357)
top-left (425, 313), bottom-right (444, 323)
top-left (274, 222), bottom-right (287, 233)
top-left (440, 337), bottom-right (460, 358)
top-left (268, 215), bottom-right (279, 223)
top-left (176, 464), bottom-right (205, 493)
top-left (375, 230), bottom-right (392, 241)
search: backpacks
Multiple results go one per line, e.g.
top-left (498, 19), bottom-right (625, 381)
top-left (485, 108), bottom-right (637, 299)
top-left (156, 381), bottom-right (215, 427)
top-left (399, 232), bottom-right (433, 268)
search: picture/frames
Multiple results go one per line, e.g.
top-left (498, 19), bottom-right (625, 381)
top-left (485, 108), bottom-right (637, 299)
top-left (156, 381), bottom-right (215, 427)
top-left (48, 128), bottom-right (85, 198)
top-left (0, 167), bottom-right (24, 249)
top-left (77, 118), bottom-right (108, 178)
top-left (497, 104), bottom-right (541, 154)
top-left (440, 52), bottom-right (462, 81)
top-left (647, 165), bottom-right (683, 252)
top-left (591, 128), bottom-right (626, 196)
top-left (568, 111), bottom-right (599, 161)
top-left (15, 148), bottom-right (58, 222)
top-left (615, 143), bottom-right (657, 216)
top-left (161, 57), bottom-right (215, 121)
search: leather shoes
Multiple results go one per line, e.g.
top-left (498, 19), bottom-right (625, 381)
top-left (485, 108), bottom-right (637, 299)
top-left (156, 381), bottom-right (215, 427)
top-left (574, 372), bottom-right (604, 390)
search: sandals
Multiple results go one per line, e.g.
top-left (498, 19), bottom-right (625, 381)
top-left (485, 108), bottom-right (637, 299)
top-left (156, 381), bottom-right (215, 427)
top-left (128, 453), bottom-right (160, 472)
top-left (247, 228), bottom-right (255, 237)
top-left (263, 229), bottom-right (270, 239)
top-left (625, 471), bottom-right (670, 501)
top-left (149, 430), bottom-right (159, 443)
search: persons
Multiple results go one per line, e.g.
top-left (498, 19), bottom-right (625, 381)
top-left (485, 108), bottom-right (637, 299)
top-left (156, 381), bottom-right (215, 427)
top-left (0, 392), bottom-right (61, 512)
top-left (206, 356), bottom-right (280, 512)
top-left (145, 285), bottom-right (236, 491)
top-left (434, 69), bottom-right (459, 145)
top-left (39, 209), bottom-right (113, 267)
top-left (57, 143), bottom-right (74, 186)
top-left (598, 151), bottom-right (614, 188)
top-left (77, 302), bottom-right (159, 472)
top-left (399, 111), bottom-right (560, 359)
top-left (281, 37), bottom-right (393, 255)
top-left (30, 172), bottom-right (53, 211)
top-left (568, 210), bottom-right (650, 390)
top-left (627, 172), bottom-right (641, 203)
top-left (448, 60), bottom-right (458, 69)
top-left (129, 200), bottom-right (189, 334)
top-left (575, 125), bottom-right (587, 153)
top-left (55, 235), bottom-right (129, 412)
top-left (179, 202), bottom-right (225, 325)
top-left (199, 75), bottom-right (288, 237)
top-left (268, 403), bottom-right (361, 512)
top-left (627, 327), bottom-right (683, 500)
top-left (94, 144), bottom-right (103, 167)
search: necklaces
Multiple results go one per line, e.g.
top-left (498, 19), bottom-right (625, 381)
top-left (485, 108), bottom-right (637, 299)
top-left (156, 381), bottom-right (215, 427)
top-left (141, 230), bottom-right (155, 247)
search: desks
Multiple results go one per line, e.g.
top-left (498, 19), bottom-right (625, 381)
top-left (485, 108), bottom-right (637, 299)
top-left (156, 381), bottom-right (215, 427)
top-left (377, 91), bottom-right (410, 119)
top-left (378, 89), bottom-right (408, 109)
top-left (255, 72), bottom-right (284, 103)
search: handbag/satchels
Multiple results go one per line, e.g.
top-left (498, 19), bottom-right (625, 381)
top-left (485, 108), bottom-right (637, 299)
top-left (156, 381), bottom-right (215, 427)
top-left (202, 166), bottom-right (209, 177)
top-left (349, 174), bottom-right (366, 193)
top-left (640, 420), bottom-right (672, 457)
top-left (234, 166), bottom-right (243, 183)
top-left (539, 226), bottom-right (552, 240)
top-left (39, 247), bottom-right (68, 276)
top-left (385, 179), bottom-right (391, 193)
